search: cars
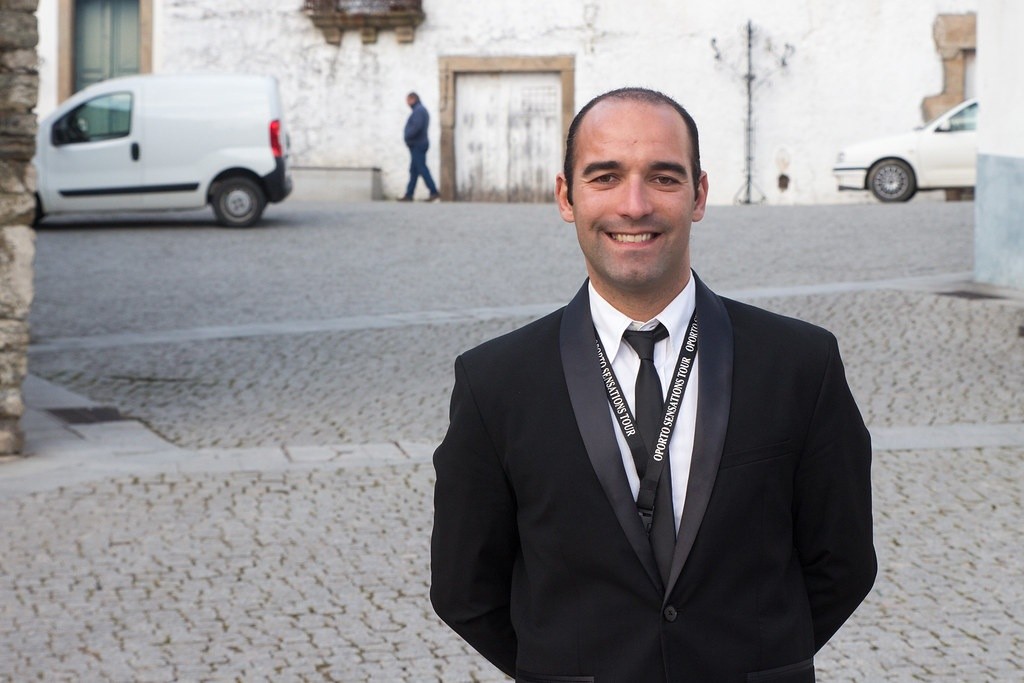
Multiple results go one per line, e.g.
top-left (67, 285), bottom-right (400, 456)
top-left (27, 70), bottom-right (296, 229)
top-left (831, 99), bottom-right (975, 204)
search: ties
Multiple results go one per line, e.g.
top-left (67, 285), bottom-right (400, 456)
top-left (622, 322), bottom-right (676, 591)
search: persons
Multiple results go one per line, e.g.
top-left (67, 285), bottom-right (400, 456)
top-left (429, 88), bottom-right (879, 683)
top-left (396, 92), bottom-right (441, 202)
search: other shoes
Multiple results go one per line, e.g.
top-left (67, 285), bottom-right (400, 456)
top-left (396, 196), bottom-right (413, 202)
top-left (423, 193), bottom-right (440, 202)
